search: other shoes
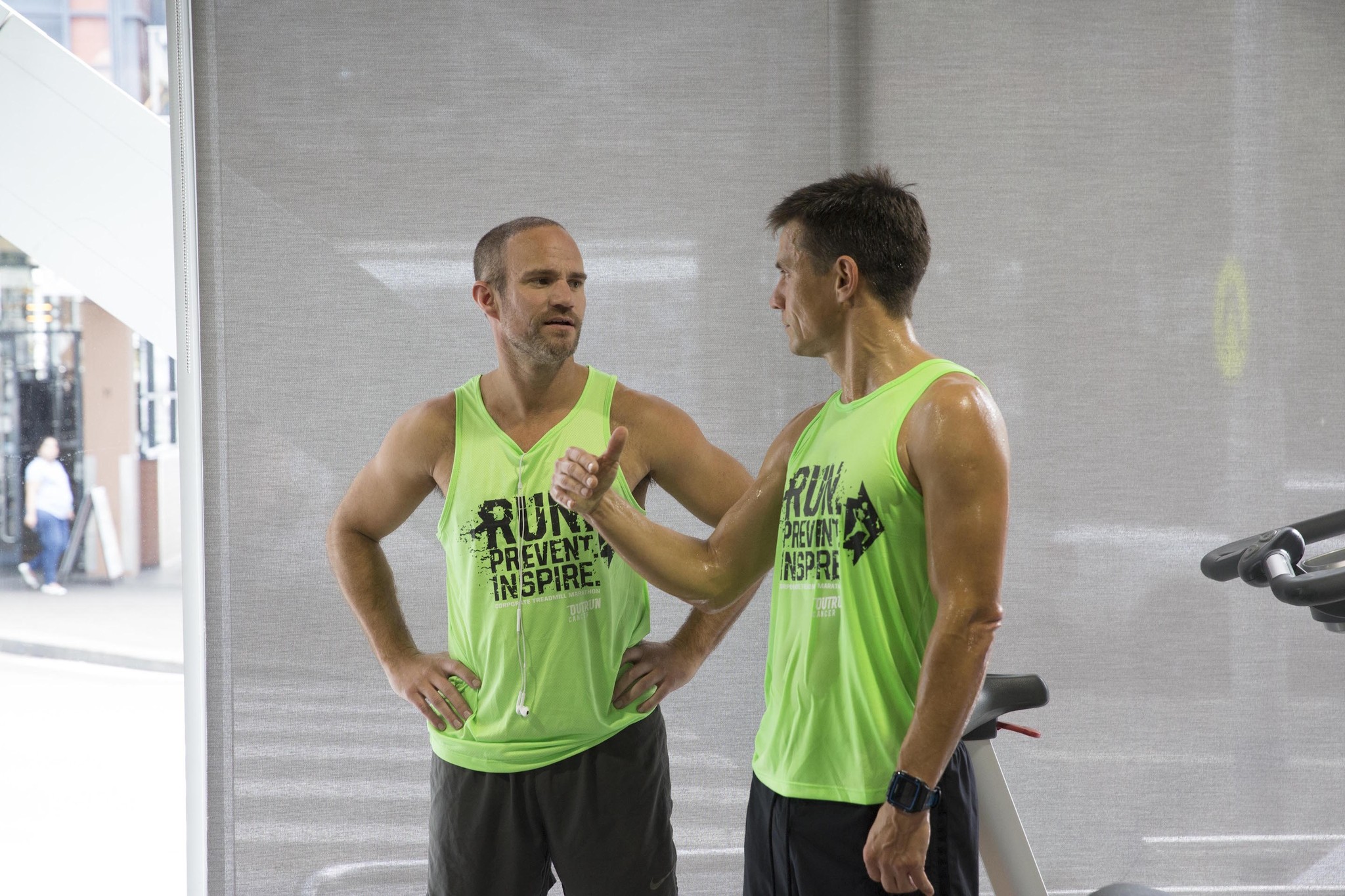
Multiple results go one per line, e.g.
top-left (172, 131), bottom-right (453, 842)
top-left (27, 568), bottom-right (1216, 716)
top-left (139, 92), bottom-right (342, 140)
top-left (42, 583), bottom-right (67, 595)
top-left (18, 562), bottom-right (39, 589)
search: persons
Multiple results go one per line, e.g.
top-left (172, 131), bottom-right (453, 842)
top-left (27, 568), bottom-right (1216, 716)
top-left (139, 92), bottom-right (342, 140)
top-left (326, 213), bottom-right (766, 896)
top-left (17, 435), bottom-right (76, 594)
top-left (551, 167), bottom-right (1009, 896)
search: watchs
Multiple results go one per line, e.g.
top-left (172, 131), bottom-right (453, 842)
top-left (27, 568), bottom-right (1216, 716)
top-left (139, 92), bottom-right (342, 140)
top-left (885, 770), bottom-right (941, 813)
